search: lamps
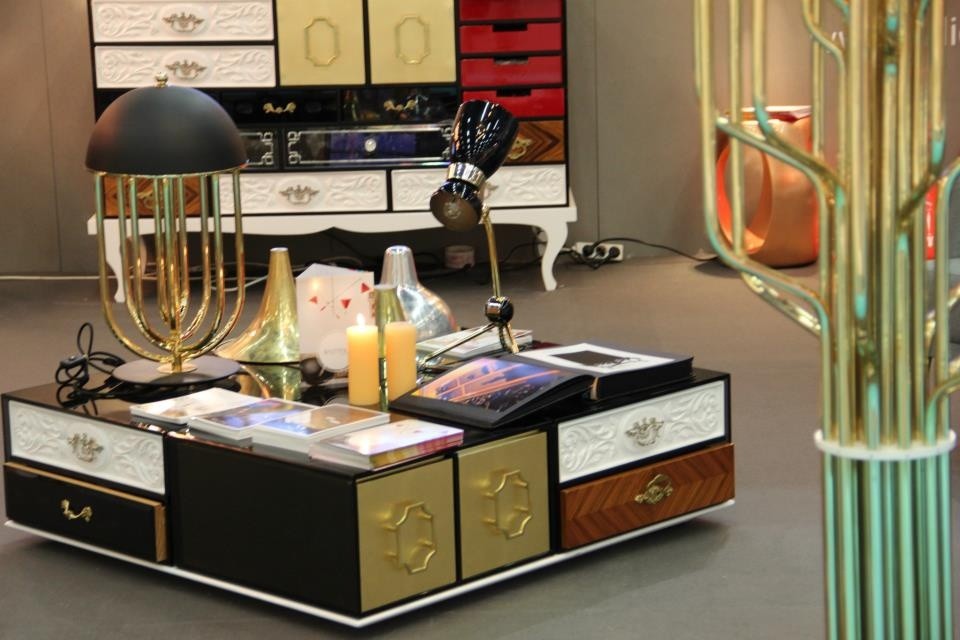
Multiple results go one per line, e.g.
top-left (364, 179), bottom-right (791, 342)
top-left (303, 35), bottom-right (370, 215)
top-left (429, 100), bottom-right (520, 368)
top-left (715, 118), bottom-right (821, 267)
top-left (296, 264), bottom-right (375, 358)
top-left (85, 76), bottom-right (251, 388)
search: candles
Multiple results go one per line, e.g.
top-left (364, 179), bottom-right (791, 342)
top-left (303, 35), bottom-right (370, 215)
top-left (348, 319), bottom-right (417, 406)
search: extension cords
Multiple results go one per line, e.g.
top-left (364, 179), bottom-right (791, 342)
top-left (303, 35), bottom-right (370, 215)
top-left (573, 241), bottom-right (624, 262)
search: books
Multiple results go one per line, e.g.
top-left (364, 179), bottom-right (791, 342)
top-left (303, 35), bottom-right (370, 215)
top-left (725, 106), bottom-right (812, 121)
top-left (129, 386), bottom-right (464, 471)
top-left (389, 342), bottom-right (696, 431)
top-left (415, 325), bottom-right (534, 358)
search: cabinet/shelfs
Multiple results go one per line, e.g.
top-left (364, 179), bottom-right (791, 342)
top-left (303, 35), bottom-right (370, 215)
top-left (87, 0), bottom-right (577, 303)
top-left (2, 326), bottom-right (735, 632)
